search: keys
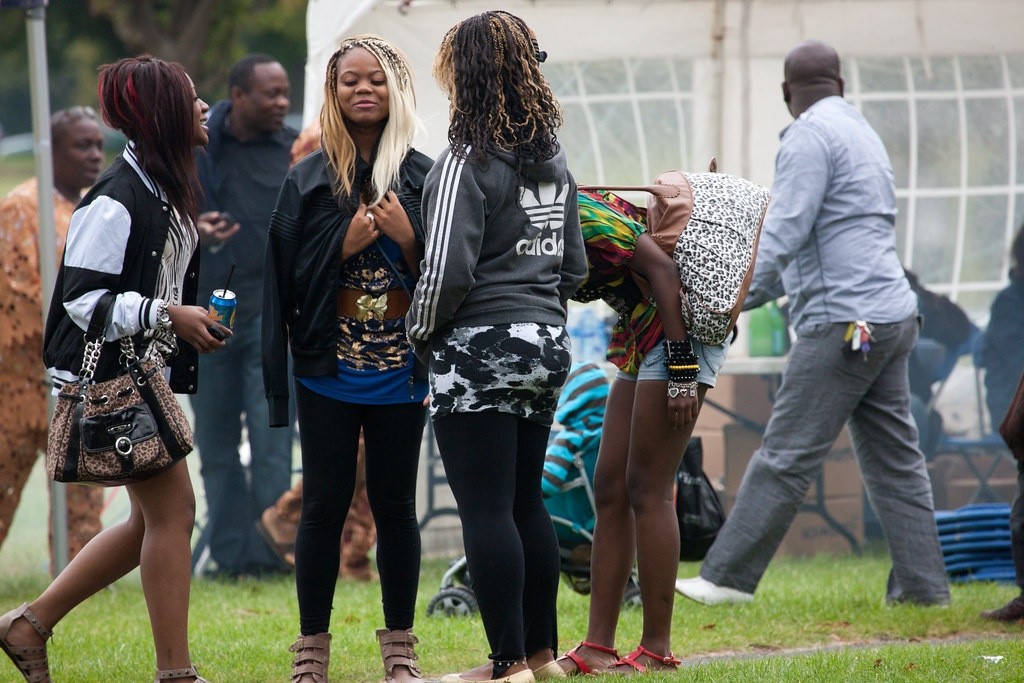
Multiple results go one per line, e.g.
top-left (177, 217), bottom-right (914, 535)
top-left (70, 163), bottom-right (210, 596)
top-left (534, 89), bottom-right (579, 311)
top-left (840, 320), bottom-right (877, 363)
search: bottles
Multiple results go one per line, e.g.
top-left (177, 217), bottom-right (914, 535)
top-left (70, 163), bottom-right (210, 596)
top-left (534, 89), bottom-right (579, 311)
top-left (748, 299), bottom-right (789, 357)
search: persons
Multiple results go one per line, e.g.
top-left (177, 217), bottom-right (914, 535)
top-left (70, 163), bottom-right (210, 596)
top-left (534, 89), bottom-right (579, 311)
top-left (0, 57), bottom-right (234, 683)
top-left (674, 41), bottom-right (950, 608)
top-left (192, 55), bottom-right (298, 588)
top-left (903, 227), bottom-right (1024, 625)
top-left (0, 106), bottom-right (106, 580)
top-left (556, 181), bottom-right (733, 678)
top-left (405, 10), bottom-right (592, 683)
top-left (255, 124), bottom-right (379, 582)
top-left (260, 36), bottom-right (436, 683)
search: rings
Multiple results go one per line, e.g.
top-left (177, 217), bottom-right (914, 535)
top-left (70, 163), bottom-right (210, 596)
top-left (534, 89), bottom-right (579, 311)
top-left (366, 213), bottom-right (374, 221)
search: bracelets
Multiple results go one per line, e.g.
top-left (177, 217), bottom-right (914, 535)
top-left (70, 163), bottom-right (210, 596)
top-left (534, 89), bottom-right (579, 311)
top-left (156, 301), bottom-right (173, 333)
top-left (663, 340), bottom-right (703, 397)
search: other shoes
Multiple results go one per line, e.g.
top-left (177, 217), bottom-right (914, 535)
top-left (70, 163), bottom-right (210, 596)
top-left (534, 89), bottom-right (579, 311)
top-left (981, 594), bottom-right (1024, 623)
top-left (439, 667), bottom-right (534, 683)
top-left (287, 633), bottom-right (332, 683)
top-left (530, 659), bottom-right (568, 681)
top-left (675, 576), bottom-right (755, 608)
top-left (375, 627), bottom-right (423, 683)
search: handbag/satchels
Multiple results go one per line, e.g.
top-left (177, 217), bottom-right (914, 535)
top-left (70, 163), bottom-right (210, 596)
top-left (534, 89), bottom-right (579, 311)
top-left (574, 159), bottom-right (772, 348)
top-left (672, 437), bottom-right (725, 564)
top-left (47, 290), bottom-right (193, 488)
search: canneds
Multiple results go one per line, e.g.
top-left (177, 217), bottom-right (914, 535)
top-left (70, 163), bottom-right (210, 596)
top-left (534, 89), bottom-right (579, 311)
top-left (208, 288), bottom-right (237, 339)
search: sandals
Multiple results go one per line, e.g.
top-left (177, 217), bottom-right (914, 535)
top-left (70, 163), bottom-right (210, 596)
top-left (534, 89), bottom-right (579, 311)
top-left (557, 641), bottom-right (621, 677)
top-left (0, 602), bottom-right (54, 683)
top-left (586, 643), bottom-right (682, 677)
top-left (153, 665), bottom-right (211, 683)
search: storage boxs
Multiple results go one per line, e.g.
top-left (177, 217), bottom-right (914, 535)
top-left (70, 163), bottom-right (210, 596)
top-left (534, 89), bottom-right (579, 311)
top-left (691, 361), bottom-right (865, 559)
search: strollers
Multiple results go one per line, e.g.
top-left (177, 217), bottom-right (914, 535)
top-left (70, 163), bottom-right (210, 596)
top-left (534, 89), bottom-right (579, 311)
top-left (425, 430), bottom-right (644, 617)
